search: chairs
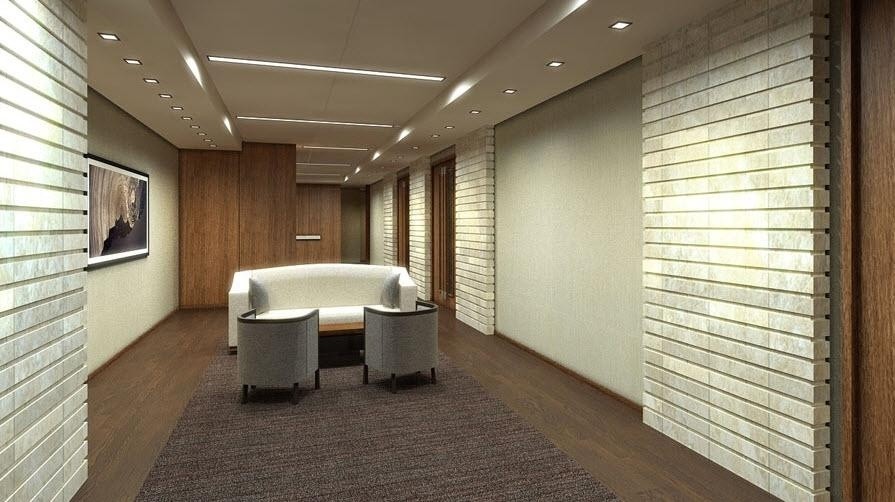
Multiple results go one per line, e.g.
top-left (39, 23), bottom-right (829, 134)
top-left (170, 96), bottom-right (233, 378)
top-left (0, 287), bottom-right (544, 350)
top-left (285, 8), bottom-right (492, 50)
top-left (361, 301), bottom-right (440, 393)
top-left (236, 308), bottom-right (322, 406)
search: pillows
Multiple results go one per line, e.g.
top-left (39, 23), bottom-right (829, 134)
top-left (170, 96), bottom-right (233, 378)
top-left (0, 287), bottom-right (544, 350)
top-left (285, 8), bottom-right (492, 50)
top-left (380, 271), bottom-right (401, 309)
top-left (249, 277), bottom-right (270, 316)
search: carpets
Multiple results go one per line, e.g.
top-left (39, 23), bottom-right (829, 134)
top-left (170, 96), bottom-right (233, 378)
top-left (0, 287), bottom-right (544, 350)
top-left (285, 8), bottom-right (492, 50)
top-left (133, 337), bottom-right (626, 502)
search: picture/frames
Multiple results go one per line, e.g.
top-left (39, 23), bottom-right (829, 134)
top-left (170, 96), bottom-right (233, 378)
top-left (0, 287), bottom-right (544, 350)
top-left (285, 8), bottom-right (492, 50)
top-left (88, 152), bottom-right (152, 269)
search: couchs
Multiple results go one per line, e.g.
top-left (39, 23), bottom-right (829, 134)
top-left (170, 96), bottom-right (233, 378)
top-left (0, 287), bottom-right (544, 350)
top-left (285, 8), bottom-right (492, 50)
top-left (227, 261), bottom-right (419, 356)
